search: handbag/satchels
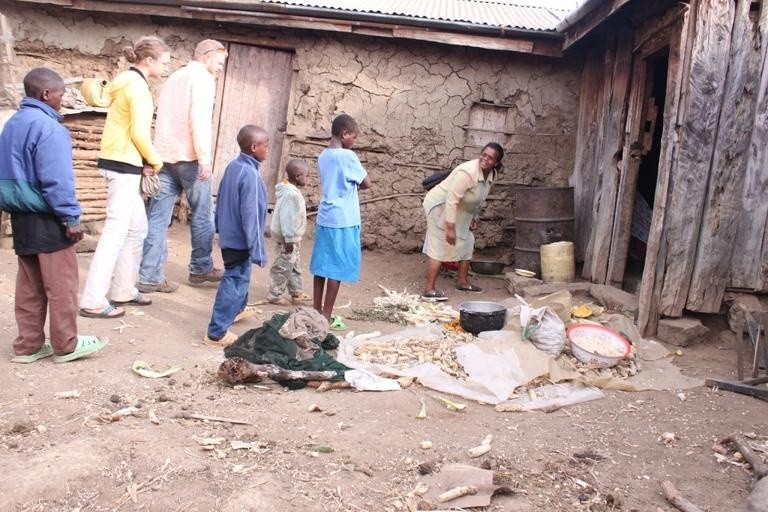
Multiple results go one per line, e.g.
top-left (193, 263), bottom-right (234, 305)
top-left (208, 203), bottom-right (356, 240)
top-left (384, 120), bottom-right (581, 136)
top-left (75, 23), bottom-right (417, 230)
top-left (421, 169), bottom-right (452, 191)
top-left (141, 165), bottom-right (160, 196)
top-left (520, 305), bottom-right (567, 359)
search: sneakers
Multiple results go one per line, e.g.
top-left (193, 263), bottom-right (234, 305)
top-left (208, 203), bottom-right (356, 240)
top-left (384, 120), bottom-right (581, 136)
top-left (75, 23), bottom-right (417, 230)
top-left (421, 291), bottom-right (449, 301)
top-left (455, 284), bottom-right (484, 293)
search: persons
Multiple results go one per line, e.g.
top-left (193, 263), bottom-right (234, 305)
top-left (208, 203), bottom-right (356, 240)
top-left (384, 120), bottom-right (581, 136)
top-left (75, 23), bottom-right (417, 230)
top-left (135, 39), bottom-right (225, 293)
top-left (203, 125), bottom-right (270, 347)
top-left (79, 35), bottom-right (172, 319)
top-left (422, 142), bottom-right (504, 301)
top-left (266, 159), bottom-right (312, 307)
top-left (0, 68), bottom-right (109, 364)
top-left (309, 114), bottom-right (371, 330)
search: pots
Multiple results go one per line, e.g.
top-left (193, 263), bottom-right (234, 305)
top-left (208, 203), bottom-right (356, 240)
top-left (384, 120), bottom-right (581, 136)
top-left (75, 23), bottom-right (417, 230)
top-left (459, 301), bottom-right (507, 333)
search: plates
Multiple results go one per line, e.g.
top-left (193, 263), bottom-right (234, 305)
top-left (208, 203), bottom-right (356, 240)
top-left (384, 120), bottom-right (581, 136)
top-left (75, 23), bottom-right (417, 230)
top-left (571, 304), bottom-right (593, 318)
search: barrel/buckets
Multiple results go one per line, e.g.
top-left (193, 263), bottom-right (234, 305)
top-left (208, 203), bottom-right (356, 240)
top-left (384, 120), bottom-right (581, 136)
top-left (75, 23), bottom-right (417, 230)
top-left (512, 184), bottom-right (576, 280)
top-left (539, 240), bottom-right (576, 284)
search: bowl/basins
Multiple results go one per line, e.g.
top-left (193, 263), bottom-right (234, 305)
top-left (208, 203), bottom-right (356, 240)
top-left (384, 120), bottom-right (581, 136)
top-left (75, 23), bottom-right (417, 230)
top-left (566, 323), bottom-right (631, 368)
top-left (515, 269), bottom-right (536, 277)
top-left (469, 261), bottom-right (505, 274)
